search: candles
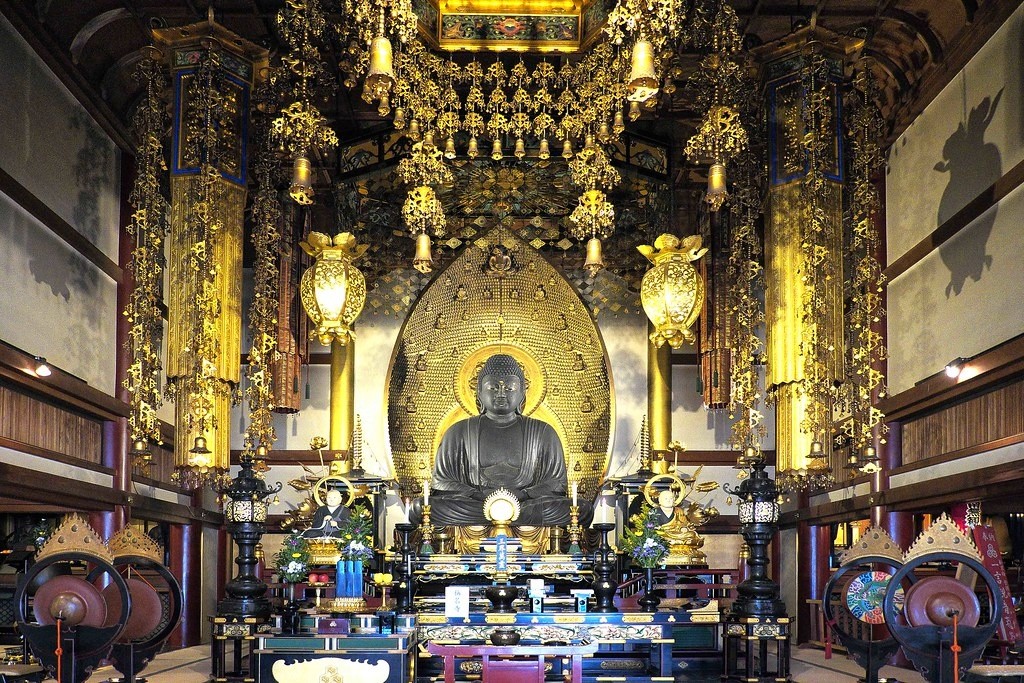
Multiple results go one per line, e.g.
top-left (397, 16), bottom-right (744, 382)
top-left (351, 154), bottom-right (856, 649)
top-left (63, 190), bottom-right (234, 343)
top-left (423, 480), bottom-right (428, 505)
top-left (572, 481), bottom-right (578, 506)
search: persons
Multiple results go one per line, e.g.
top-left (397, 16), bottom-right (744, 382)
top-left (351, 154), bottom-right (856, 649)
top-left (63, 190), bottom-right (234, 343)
top-left (296, 489), bottom-right (356, 538)
top-left (394, 352), bottom-right (595, 529)
top-left (644, 490), bottom-right (698, 541)
top-left (391, 240), bottom-right (606, 496)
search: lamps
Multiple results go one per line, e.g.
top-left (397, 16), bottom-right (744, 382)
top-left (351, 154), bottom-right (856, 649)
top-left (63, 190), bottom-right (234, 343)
top-left (274, 0), bottom-right (752, 346)
top-left (944, 356), bottom-right (973, 376)
top-left (34, 356), bottom-right (51, 376)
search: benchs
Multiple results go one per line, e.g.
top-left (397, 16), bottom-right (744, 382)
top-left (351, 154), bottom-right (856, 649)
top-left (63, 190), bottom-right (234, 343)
top-left (0, 664), bottom-right (44, 683)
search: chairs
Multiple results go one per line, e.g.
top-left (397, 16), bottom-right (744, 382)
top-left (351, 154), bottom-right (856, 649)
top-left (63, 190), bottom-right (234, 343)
top-left (425, 639), bottom-right (600, 683)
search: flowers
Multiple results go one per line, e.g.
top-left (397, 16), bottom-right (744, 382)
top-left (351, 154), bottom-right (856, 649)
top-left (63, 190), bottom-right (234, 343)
top-left (270, 529), bottom-right (315, 583)
top-left (331, 500), bottom-right (374, 567)
